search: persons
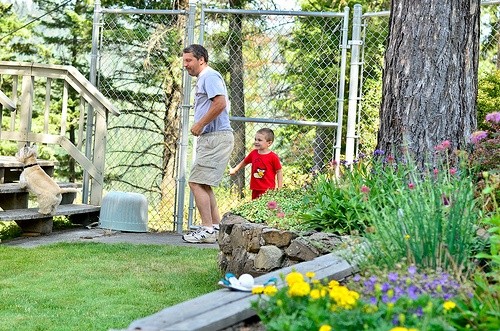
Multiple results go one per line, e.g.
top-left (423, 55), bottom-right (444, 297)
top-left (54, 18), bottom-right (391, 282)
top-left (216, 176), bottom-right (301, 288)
top-left (182, 44), bottom-right (235, 244)
top-left (229, 127), bottom-right (283, 200)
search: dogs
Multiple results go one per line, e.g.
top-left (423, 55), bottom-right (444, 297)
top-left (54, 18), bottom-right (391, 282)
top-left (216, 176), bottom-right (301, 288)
top-left (15, 142), bottom-right (63, 215)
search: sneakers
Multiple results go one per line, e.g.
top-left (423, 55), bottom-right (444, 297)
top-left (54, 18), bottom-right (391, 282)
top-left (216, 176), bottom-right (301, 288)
top-left (181, 227), bottom-right (217, 245)
top-left (212, 223), bottom-right (221, 240)
